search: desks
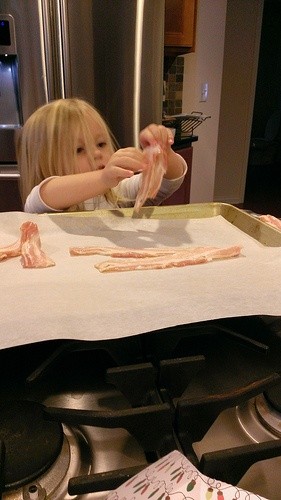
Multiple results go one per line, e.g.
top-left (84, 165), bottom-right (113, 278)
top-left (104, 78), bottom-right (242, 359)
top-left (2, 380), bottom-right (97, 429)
top-left (0, 201), bottom-right (281, 350)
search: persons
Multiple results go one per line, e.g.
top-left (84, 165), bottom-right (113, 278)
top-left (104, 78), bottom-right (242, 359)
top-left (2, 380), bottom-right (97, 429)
top-left (14, 97), bottom-right (188, 214)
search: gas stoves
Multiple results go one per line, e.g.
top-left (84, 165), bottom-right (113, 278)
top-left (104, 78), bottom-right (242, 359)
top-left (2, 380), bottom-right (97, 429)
top-left (0, 349), bottom-right (281, 500)
